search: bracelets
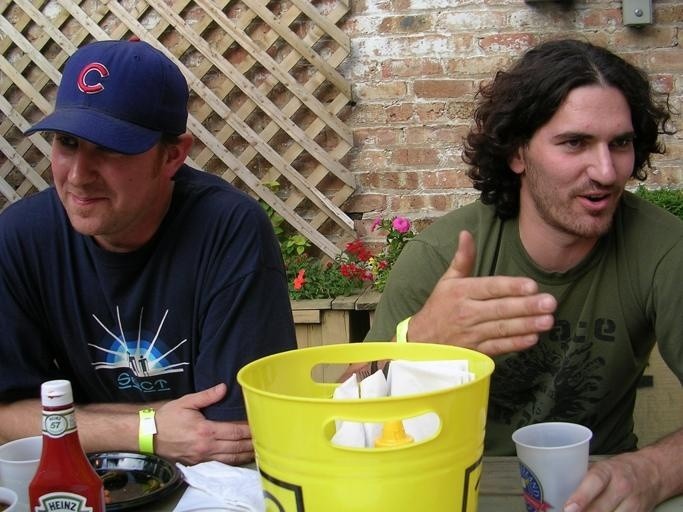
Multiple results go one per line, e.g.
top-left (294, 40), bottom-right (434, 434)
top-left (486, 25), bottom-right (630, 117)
top-left (139, 409), bottom-right (157, 455)
top-left (396, 316), bottom-right (409, 342)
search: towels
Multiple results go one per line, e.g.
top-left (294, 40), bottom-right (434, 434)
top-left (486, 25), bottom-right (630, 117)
top-left (331, 357), bottom-right (475, 449)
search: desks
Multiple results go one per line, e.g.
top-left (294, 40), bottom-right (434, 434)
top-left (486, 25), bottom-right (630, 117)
top-left (227, 448), bottom-right (681, 511)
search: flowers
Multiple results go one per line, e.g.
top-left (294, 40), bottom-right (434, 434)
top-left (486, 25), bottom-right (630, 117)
top-left (284, 234), bottom-right (373, 297)
top-left (365, 209), bottom-right (418, 291)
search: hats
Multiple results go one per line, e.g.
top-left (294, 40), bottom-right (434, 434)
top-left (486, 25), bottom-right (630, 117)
top-left (23, 40), bottom-right (187, 155)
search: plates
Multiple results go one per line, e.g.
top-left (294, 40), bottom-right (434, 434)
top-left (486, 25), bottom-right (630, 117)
top-left (81, 447), bottom-right (186, 511)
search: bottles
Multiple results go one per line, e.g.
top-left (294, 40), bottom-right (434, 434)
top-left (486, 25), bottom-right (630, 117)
top-left (27, 380), bottom-right (106, 512)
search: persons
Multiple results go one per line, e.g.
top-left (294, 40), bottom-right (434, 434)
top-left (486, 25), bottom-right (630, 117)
top-left (0, 35), bottom-right (299, 467)
top-left (337, 42), bottom-right (683, 512)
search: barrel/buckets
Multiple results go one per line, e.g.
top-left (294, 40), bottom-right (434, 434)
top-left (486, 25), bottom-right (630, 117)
top-left (237, 342), bottom-right (496, 512)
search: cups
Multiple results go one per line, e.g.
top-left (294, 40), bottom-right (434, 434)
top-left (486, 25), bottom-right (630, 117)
top-left (509, 422), bottom-right (595, 511)
top-left (0, 434), bottom-right (48, 512)
